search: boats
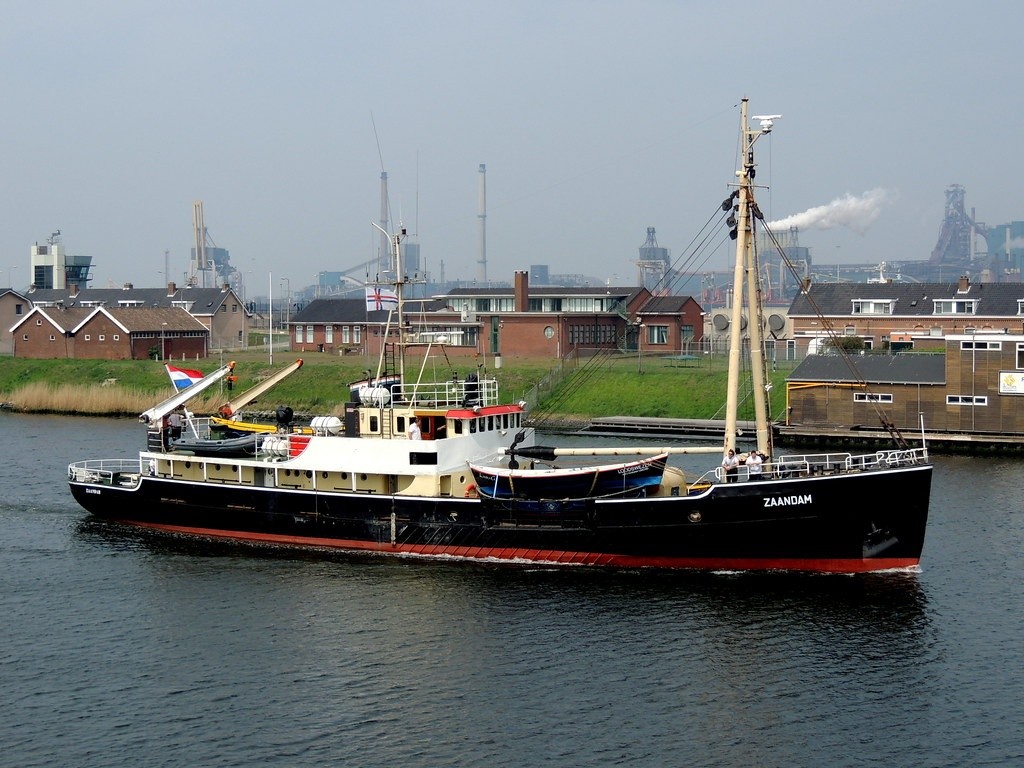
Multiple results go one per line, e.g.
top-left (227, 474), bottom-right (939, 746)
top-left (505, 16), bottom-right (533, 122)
top-left (66, 100), bottom-right (933, 580)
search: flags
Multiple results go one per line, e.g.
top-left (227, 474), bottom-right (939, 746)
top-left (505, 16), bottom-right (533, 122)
top-left (168, 365), bottom-right (205, 388)
top-left (367, 288), bottom-right (399, 312)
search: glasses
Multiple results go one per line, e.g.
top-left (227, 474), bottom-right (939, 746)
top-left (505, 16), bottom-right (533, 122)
top-left (729, 453), bottom-right (733, 455)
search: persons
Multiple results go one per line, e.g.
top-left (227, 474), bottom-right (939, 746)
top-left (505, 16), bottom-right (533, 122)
top-left (721, 449), bottom-right (739, 483)
top-left (408, 418), bottom-right (422, 440)
top-left (745, 451), bottom-right (762, 482)
top-left (167, 409), bottom-right (182, 441)
top-left (221, 404), bottom-right (232, 420)
top-left (461, 373), bottom-right (484, 407)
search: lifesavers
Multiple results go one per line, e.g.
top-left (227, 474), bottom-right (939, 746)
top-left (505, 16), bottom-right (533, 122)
top-left (465, 484), bottom-right (479, 498)
